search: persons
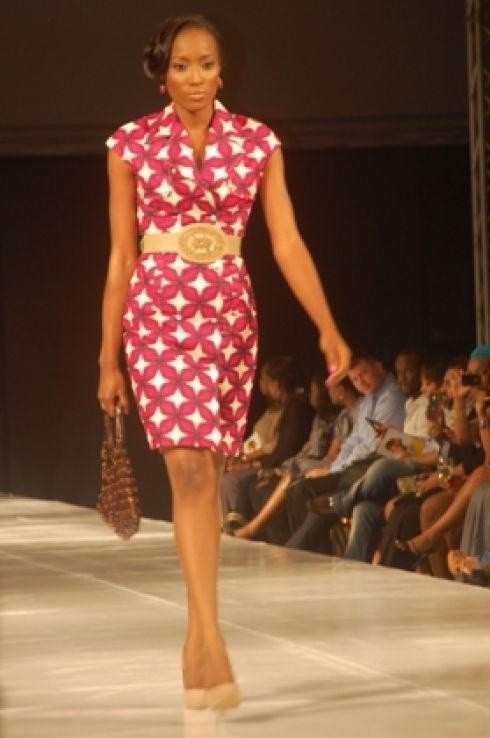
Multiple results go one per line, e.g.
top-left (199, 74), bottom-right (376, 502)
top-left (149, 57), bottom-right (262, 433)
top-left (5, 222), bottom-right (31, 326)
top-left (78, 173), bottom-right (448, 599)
top-left (93, 13), bottom-right (356, 719)
top-left (206, 337), bottom-right (489, 589)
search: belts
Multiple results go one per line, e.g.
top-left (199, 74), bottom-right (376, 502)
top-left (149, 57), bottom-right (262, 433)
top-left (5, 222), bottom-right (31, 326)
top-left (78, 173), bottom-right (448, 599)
top-left (138, 222), bottom-right (244, 266)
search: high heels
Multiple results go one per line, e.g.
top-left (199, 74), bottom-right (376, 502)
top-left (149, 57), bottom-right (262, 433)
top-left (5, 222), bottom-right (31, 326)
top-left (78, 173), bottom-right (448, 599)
top-left (205, 678), bottom-right (244, 713)
top-left (177, 639), bottom-right (210, 710)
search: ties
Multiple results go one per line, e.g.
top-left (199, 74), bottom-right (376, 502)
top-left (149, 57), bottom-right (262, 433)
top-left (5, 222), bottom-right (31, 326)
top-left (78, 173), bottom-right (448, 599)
top-left (369, 395), bottom-right (378, 417)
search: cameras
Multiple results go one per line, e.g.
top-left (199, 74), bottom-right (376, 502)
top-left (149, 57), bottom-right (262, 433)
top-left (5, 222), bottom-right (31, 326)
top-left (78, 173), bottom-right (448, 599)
top-left (462, 374), bottom-right (480, 386)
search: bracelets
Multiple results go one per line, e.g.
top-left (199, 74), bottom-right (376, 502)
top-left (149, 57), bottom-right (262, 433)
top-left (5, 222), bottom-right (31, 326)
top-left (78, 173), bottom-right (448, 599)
top-left (451, 410), bottom-right (465, 418)
top-left (478, 416), bottom-right (489, 430)
top-left (241, 455), bottom-right (247, 463)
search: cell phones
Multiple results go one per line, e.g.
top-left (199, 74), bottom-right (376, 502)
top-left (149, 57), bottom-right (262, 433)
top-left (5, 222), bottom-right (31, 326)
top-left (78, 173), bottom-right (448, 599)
top-left (366, 418), bottom-right (381, 429)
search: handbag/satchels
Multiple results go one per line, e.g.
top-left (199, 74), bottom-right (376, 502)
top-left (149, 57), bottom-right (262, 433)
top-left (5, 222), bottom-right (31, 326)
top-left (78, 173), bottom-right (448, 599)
top-left (91, 401), bottom-right (144, 544)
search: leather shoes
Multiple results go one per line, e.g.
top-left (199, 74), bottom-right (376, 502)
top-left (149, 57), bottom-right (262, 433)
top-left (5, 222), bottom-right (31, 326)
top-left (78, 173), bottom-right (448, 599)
top-left (221, 491), bottom-right (490, 586)
